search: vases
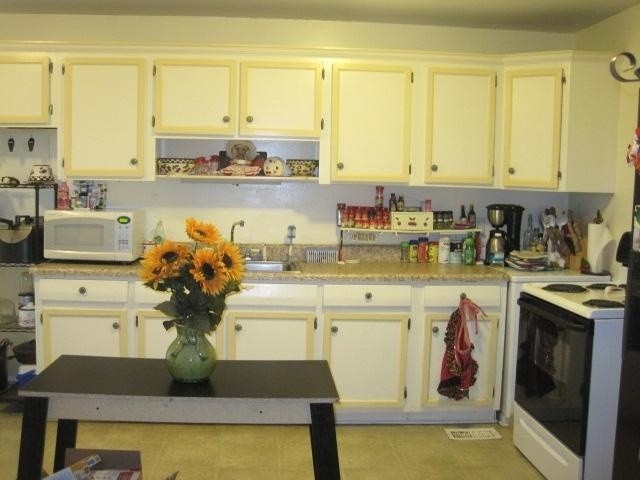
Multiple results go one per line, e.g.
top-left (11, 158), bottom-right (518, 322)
top-left (164, 317), bottom-right (217, 383)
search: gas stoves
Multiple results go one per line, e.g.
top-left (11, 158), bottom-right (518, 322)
top-left (522, 280), bottom-right (625, 319)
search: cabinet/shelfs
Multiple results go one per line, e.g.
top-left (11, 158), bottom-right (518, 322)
top-left (318, 277), bottom-right (417, 426)
top-left (418, 51), bottom-right (502, 188)
top-left (327, 46), bottom-right (419, 188)
top-left (131, 273), bottom-right (225, 364)
top-left (57, 44), bottom-right (154, 179)
top-left (149, 43), bottom-right (327, 141)
top-left (224, 277), bottom-right (318, 363)
top-left (27, 269), bottom-right (131, 373)
top-left (0, 38), bottom-right (60, 132)
top-left (416, 277), bottom-right (507, 424)
top-left (0, 183), bottom-right (56, 399)
top-left (503, 54), bottom-right (619, 194)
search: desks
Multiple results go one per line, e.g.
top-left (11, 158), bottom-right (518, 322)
top-left (18, 354), bottom-right (341, 480)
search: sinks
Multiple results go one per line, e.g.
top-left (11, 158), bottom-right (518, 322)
top-left (242, 260), bottom-right (303, 272)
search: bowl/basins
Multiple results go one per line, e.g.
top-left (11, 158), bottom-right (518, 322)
top-left (157, 156), bottom-right (197, 174)
top-left (286, 158), bottom-right (319, 176)
top-left (2, 176), bottom-right (19, 187)
top-left (27, 165), bottom-right (54, 182)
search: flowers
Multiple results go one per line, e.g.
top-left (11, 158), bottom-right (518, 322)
top-left (134, 215), bottom-right (246, 336)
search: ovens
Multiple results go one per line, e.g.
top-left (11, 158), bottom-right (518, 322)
top-left (513, 290), bottom-right (595, 480)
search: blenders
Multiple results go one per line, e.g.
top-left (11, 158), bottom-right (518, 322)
top-left (484, 204), bottom-right (525, 267)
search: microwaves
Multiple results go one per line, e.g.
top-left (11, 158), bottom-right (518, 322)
top-left (43, 209), bottom-right (145, 263)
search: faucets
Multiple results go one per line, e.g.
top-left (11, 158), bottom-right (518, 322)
top-left (231, 220), bottom-right (244, 245)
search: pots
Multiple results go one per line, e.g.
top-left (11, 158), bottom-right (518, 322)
top-left (13, 339), bottom-right (36, 364)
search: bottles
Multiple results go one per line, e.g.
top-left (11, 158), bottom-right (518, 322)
top-left (433, 210), bottom-right (453, 230)
top-left (437, 237), bottom-right (450, 264)
top-left (57, 178), bottom-right (70, 210)
top-left (417, 237), bottom-right (428, 263)
top-left (375, 186), bottom-right (384, 208)
top-left (475, 231), bottom-right (482, 262)
top-left (425, 200), bottom-right (431, 212)
top-left (449, 242), bottom-right (462, 264)
top-left (529, 228), bottom-right (540, 252)
top-left (460, 204), bottom-right (467, 225)
top-left (467, 204), bottom-right (476, 227)
top-left (396, 196), bottom-right (405, 211)
top-left (427, 242), bottom-right (439, 264)
top-left (477, 207), bottom-right (485, 231)
top-left (409, 239), bottom-right (418, 263)
top-left (535, 232), bottom-right (545, 252)
top-left (389, 193), bottom-right (396, 211)
top-left (400, 239), bottom-right (410, 263)
top-left (523, 213), bottom-right (533, 250)
top-left (464, 232), bottom-right (475, 265)
top-left (335, 203), bottom-right (392, 229)
